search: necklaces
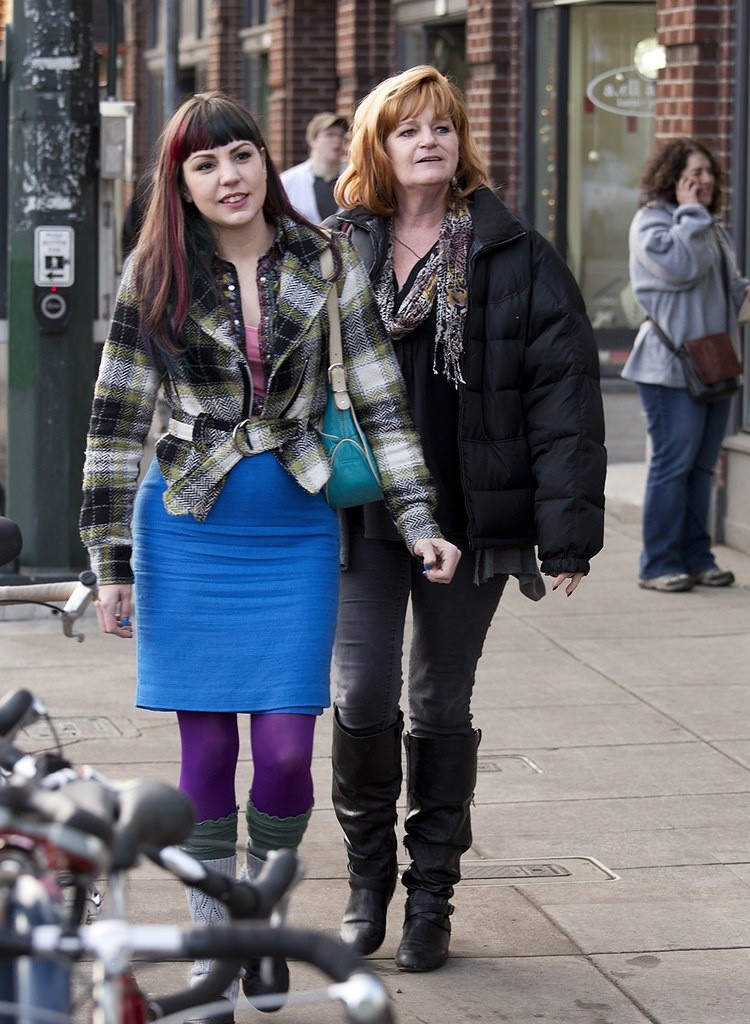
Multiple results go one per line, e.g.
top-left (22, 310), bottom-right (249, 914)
top-left (392, 234), bottom-right (422, 260)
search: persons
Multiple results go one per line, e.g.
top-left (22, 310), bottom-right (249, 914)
top-left (619, 139), bottom-right (750, 595)
top-left (79, 89), bottom-right (461, 1024)
top-left (279, 108), bottom-right (357, 225)
top-left (316, 62), bottom-right (608, 1024)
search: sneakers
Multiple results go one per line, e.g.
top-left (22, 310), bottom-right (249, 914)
top-left (639, 573), bottom-right (694, 591)
top-left (695, 566), bottom-right (734, 586)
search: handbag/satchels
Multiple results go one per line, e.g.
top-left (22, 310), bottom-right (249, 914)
top-left (676, 332), bottom-right (745, 401)
top-left (320, 228), bottom-right (386, 511)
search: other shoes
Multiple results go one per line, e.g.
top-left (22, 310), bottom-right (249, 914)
top-left (243, 951), bottom-right (290, 1012)
top-left (181, 997), bottom-right (235, 1024)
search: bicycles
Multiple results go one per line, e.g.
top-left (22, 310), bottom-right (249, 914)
top-left (0, 516), bottom-right (397, 1023)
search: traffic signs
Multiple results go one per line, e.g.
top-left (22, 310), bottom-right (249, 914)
top-left (32, 224), bottom-right (76, 288)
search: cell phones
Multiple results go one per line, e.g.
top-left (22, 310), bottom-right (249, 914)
top-left (668, 172), bottom-right (702, 198)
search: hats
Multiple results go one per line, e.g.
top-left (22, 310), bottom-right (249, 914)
top-left (306, 114), bottom-right (349, 142)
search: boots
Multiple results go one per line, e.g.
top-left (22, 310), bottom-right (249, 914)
top-left (332, 703), bottom-right (405, 957)
top-left (394, 729), bottom-right (483, 972)
